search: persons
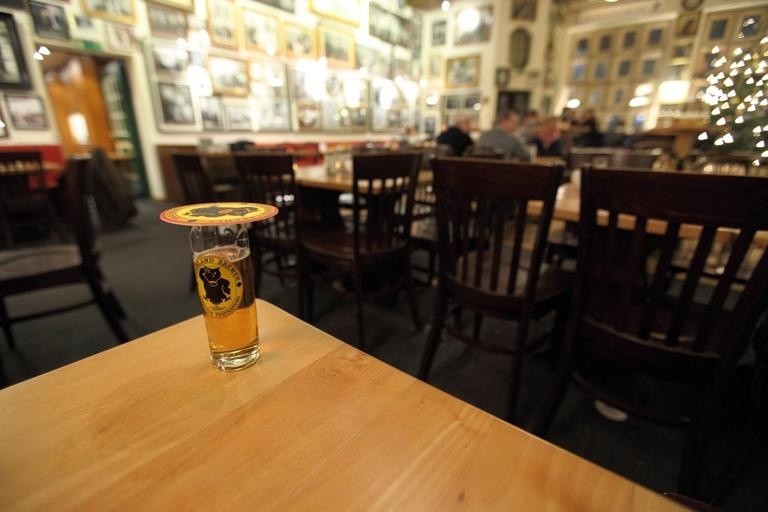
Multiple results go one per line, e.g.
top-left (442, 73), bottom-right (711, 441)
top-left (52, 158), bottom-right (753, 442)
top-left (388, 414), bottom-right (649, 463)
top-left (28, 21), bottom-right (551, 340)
top-left (434, 106), bottom-right (658, 250)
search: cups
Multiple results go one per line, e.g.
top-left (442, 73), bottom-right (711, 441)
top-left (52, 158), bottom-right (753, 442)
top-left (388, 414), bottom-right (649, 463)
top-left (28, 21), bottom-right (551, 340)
top-left (190, 226), bottom-right (261, 371)
